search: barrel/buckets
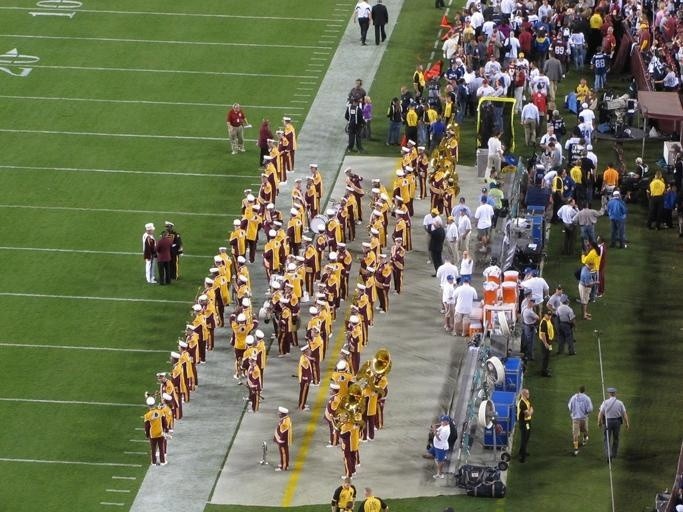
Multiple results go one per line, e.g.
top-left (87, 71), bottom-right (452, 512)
top-left (483, 270), bottom-right (519, 305)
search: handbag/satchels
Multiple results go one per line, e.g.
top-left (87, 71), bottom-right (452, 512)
top-left (574, 267), bottom-right (581, 280)
top-left (454, 464), bottom-right (506, 497)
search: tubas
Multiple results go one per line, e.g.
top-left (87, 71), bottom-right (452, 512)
top-left (332, 349), bottom-right (391, 429)
top-left (433, 121), bottom-right (460, 196)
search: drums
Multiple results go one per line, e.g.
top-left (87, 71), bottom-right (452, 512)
top-left (259, 199), bottom-right (338, 322)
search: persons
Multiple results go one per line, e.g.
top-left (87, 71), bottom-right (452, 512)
top-left (142, 1), bottom-right (683, 512)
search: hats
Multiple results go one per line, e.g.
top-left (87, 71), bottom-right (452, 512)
top-left (522, 267), bottom-right (569, 315)
top-left (460, 182), bottom-right (497, 282)
top-left (326, 167), bottom-right (412, 218)
top-left (244, 116), bottom-right (318, 216)
top-left (402, 124), bottom-right (456, 154)
top-left (145, 222), bottom-right (455, 414)
top-left (607, 388), bottom-right (616, 393)
top-left (439, 416), bottom-right (450, 421)
